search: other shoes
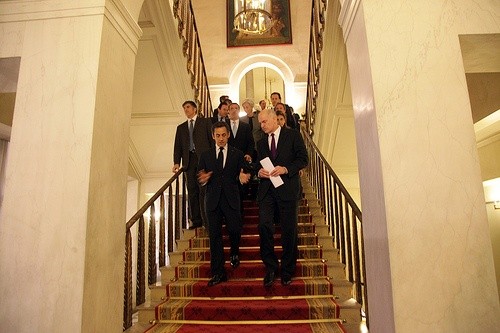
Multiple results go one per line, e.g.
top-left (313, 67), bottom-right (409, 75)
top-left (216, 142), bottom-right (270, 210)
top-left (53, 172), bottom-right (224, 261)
top-left (189, 223), bottom-right (200, 229)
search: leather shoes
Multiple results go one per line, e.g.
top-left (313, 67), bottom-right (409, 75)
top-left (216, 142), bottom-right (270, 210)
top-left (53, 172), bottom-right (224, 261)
top-left (208, 273), bottom-right (228, 286)
top-left (264, 272), bottom-right (277, 286)
top-left (230, 255), bottom-right (240, 266)
top-left (281, 273), bottom-right (292, 285)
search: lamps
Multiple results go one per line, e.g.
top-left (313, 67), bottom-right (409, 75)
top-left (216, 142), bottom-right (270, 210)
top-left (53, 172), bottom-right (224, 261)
top-left (232, 0), bottom-right (278, 36)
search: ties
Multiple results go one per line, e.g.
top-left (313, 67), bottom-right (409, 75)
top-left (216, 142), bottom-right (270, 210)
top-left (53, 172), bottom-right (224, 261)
top-left (220, 116), bottom-right (223, 121)
top-left (270, 133), bottom-right (276, 159)
top-left (233, 121), bottom-right (237, 137)
top-left (189, 119), bottom-right (194, 152)
top-left (217, 147), bottom-right (224, 168)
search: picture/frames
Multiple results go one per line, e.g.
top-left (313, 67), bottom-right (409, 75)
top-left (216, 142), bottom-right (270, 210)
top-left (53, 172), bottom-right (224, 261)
top-left (226, 0), bottom-right (293, 49)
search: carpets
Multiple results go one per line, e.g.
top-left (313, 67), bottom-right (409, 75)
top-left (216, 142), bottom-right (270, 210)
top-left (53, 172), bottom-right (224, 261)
top-left (144, 179), bottom-right (350, 333)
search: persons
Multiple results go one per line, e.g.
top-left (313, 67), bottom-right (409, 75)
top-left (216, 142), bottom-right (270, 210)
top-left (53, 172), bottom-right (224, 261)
top-left (251, 107), bottom-right (310, 288)
top-left (171, 101), bottom-right (214, 230)
top-left (209, 91), bottom-right (305, 198)
top-left (202, 122), bottom-right (252, 288)
